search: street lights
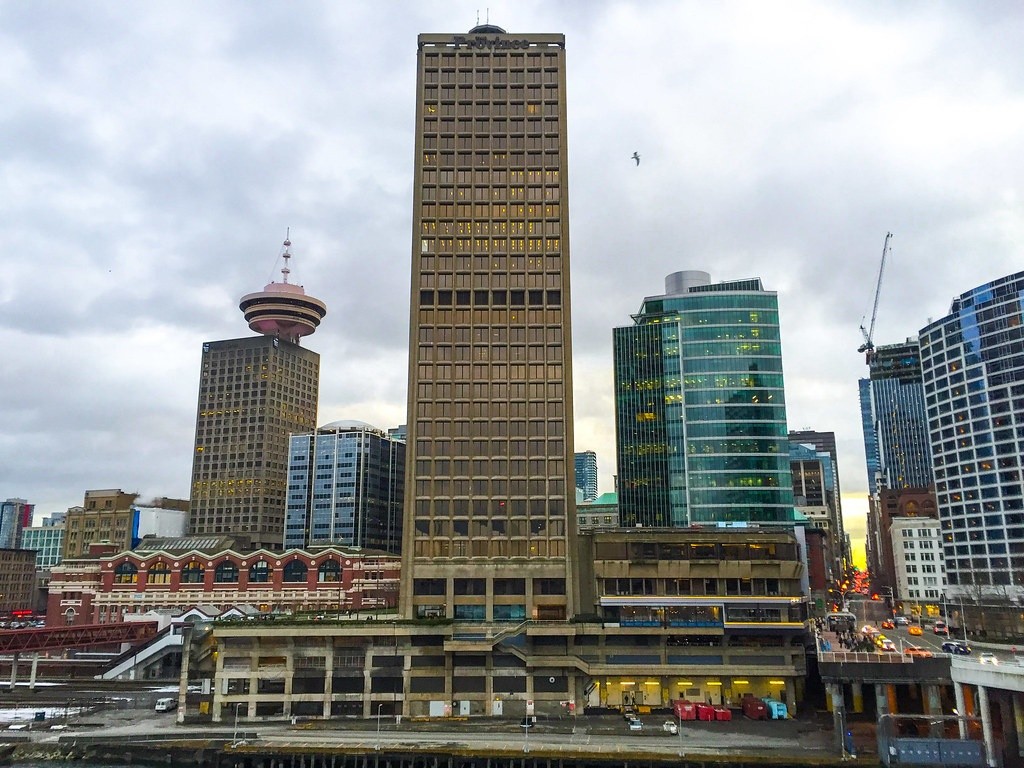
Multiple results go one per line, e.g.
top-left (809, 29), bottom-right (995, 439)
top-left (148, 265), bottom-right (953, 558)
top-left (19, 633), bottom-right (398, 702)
top-left (828, 589), bottom-right (855, 613)
top-left (234, 702), bottom-right (243, 745)
top-left (377, 704), bottom-right (382, 750)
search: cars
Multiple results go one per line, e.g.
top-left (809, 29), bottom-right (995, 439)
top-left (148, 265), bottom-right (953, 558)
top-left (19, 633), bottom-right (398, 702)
top-left (909, 627), bottom-right (922, 636)
top-left (979, 652), bottom-right (997, 665)
top-left (942, 642), bottom-right (971, 655)
top-left (862, 625), bottom-right (894, 651)
top-left (521, 717), bottom-right (535, 728)
top-left (852, 573), bottom-right (869, 595)
top-left (663, 722), bottom-right (677, 735)
top-left (0, 620), bottom-right (45, 629)
top-left (882, 617), bottom-right (912, 629)
top-left (905, 647), bottom-right (932, 658)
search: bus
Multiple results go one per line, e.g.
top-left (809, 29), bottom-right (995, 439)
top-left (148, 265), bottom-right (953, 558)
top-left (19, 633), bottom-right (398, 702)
top-left (934, 621), bottom-right (947, 635)
top-left (826, 611), bottom-right (857, 636)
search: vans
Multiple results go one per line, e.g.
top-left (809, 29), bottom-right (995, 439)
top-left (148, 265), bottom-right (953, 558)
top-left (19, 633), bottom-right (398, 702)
top-left (628, 716), bottom-right (643, 731)
top-left (623, 709), bottom-right (636, 720)
top-left (155, 698), bottom-right (177, 713)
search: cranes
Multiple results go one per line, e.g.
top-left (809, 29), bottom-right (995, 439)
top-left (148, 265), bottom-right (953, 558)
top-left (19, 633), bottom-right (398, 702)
top-left (858, 230), bottom-right (893, 365)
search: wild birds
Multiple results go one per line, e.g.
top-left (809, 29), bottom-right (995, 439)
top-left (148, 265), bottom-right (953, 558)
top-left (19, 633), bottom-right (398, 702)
top-left (631, 151), bottom-right (641, 166)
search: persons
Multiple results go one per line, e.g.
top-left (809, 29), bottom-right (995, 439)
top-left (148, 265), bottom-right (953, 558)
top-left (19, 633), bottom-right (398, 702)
top-left (348, 609), bottom-right (352, 618)
top-left (815, 616), bottom-right (898, 652)
top-left (624, 695), bottom-right (637, 705)
